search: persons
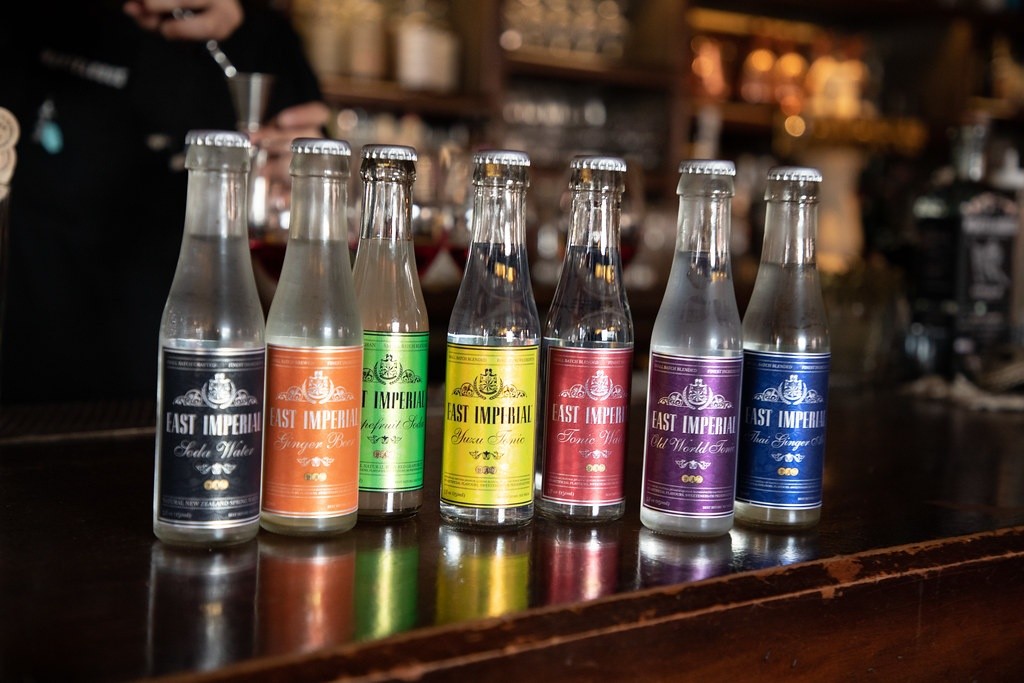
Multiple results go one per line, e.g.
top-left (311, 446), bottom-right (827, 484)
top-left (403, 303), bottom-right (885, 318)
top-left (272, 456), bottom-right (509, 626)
top-left (0, 1), bottom-right (335, 438)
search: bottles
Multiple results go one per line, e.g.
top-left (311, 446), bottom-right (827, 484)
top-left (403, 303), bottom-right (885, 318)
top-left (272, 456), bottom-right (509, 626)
top-left (734, 168), bottom-right (831, 531)
top-left (153, 130), bottom-right (267, 548)
top-left (261, 137), bottom-right (363, 535)
top-left (640, 160), bottom-right (743, 538)
top-left (535, 155), bottom-right (633, 525)
top-left (350, 145), bottom-right (429, 525)
top-left (438, 151), bottom-right (541, 535)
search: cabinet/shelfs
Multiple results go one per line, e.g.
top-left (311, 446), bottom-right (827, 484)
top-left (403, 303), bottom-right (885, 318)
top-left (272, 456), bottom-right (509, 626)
top-left (259, 0), bottom-right (830, 248)
